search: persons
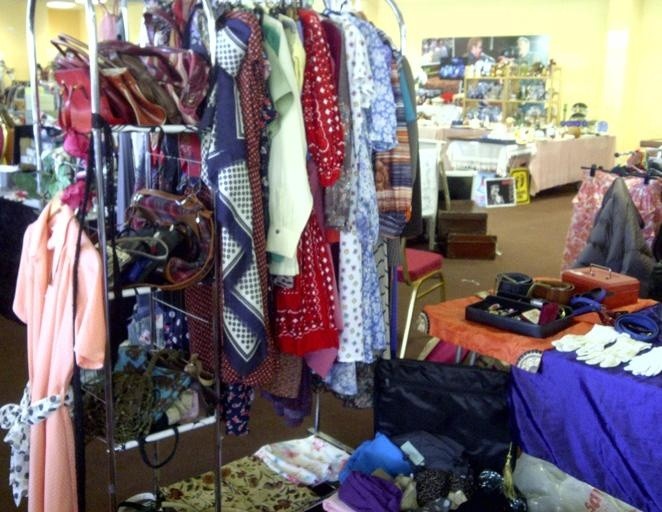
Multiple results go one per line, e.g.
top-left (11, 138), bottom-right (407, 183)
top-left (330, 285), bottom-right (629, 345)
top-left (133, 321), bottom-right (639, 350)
top-left (490, 181), bottom-right (504, 206)
top-left (461, 38), bottom-right (486, 62)
top-left (497, 37), bottom-right (537, 67)
top-left (422, 39), bottom-right (448, 63)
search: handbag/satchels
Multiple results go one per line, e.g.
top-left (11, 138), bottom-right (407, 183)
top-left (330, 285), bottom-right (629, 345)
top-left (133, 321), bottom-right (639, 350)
top-left (52, 34), bottom-right (205, 133)
top-left (95, 188), bottom-right (210, 288)
top-left (79, 344), bottom-right (200, 442)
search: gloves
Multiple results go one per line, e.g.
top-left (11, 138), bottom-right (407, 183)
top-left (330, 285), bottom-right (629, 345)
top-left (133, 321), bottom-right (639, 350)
top-left (551, 323), bottom-right (661, 376)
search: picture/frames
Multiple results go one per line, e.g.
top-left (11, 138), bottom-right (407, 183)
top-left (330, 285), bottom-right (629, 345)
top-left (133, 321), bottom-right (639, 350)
top-left (484, 177), bottom-right (516, 209)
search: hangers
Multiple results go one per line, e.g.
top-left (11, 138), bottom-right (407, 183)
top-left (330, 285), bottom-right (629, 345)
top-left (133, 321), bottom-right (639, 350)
top-left (48, 162), bottom-right (85, 220)
top-left (230, 0), bottom-right (355, 26)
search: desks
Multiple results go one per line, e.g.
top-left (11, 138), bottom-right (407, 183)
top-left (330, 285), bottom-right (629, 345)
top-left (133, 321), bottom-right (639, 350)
top-left (419, 134), bottom-right (616, 198)
top-left (419, 277), bottom-right (662, 408)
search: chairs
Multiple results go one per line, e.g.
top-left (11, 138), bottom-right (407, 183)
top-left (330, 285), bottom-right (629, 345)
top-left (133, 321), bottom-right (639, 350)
top-left (396, 236), bottom-right (447, 359)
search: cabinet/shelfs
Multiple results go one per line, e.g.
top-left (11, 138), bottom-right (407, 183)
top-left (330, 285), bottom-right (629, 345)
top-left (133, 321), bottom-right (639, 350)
top-left (27, 0), bottom-right (224, 512)
top-left (462, 75), bottom-right (561, 129)
